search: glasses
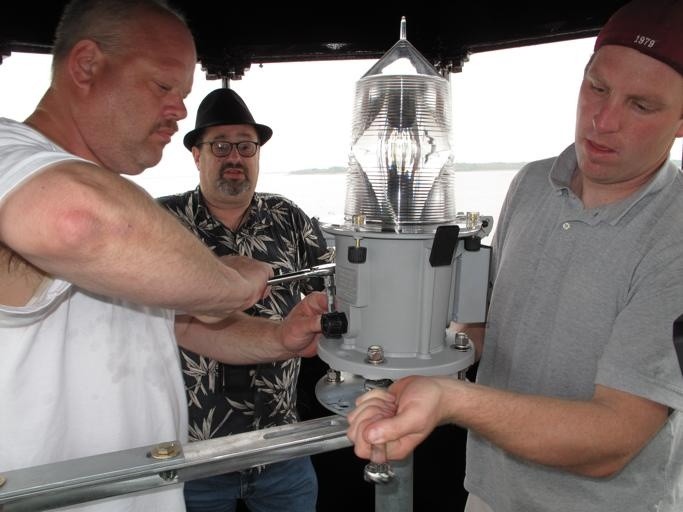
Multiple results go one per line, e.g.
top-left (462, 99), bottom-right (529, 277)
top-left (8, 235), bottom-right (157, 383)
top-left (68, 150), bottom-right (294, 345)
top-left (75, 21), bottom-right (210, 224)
top-left (196, 141), bottom-right (261, 157)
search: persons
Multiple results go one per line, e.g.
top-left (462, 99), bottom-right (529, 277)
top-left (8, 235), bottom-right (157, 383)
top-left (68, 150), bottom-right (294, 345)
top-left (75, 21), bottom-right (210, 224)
top-left (347, 0), bottom-right (683, 512)
top-left (154, 88), bottom-right (336, 512)
top-left (0, 0), bottom-right (329, 512)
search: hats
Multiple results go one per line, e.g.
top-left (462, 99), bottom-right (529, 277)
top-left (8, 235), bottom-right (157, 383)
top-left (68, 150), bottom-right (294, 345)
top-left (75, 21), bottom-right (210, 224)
top-left (594, 2), bottom-right (683, 77)
top-left (183, 89), bottom-right (274, 152)
